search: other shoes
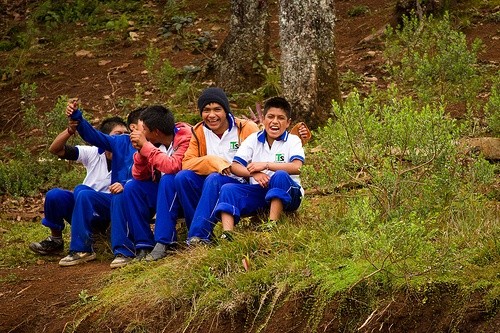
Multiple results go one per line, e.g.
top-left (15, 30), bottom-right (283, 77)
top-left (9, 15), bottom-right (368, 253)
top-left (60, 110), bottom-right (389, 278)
top-left (263, 220), bottom-right (279, 232)
top-left (145, 243), bottom-right (168, 262)
top-left (218, 231), bottom-right (238, 242)
top-left (133, 249), bottom-right (150, 262)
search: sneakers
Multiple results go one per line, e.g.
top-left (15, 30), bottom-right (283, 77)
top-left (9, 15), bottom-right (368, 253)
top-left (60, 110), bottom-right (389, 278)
top-left (59, 251), bottom-right (96, 266)
top-left (29, 236), bottom-right (64, 254)
top-left (109, 253), bottom-right (135, 268)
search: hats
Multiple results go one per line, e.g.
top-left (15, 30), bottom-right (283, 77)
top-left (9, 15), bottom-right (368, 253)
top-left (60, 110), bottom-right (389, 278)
top-left (197, 87), bottom-right (230, 117)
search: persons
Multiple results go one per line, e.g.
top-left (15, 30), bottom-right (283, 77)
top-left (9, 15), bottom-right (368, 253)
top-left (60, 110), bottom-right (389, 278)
top-left (28, 115), bottom-right (129, 255)
top-left (214, 96), bottom-right (305, 241)
top-left (175, 89), bottom-right (311, 246)
top-left (124, 106), bottom-right (193, 262)
top-left (59, 101), bottom-right (149, 268)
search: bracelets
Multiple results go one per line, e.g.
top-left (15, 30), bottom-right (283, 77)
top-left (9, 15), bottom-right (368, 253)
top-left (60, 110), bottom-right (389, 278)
top-left (267, 162), bottom-right (269, 170)
top-left (68, 127), bottom-right (76, 137)
